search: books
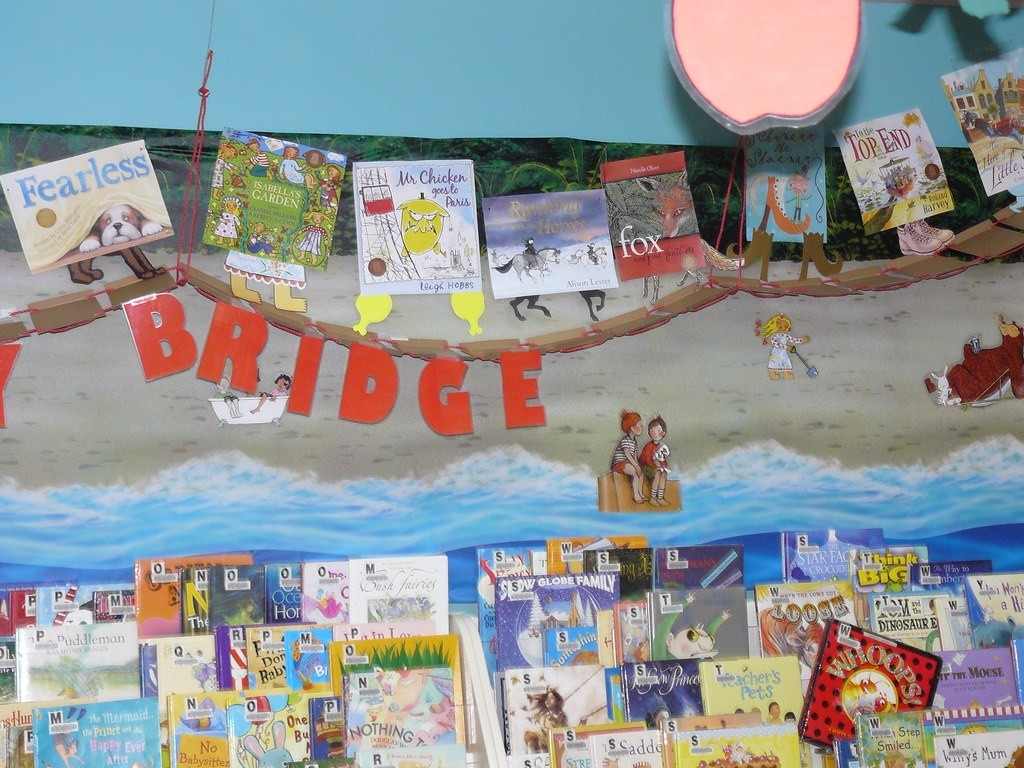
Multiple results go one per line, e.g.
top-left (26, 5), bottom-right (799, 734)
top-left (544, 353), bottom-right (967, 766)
top-left (0, 527), bottom-right (1024, 768)
